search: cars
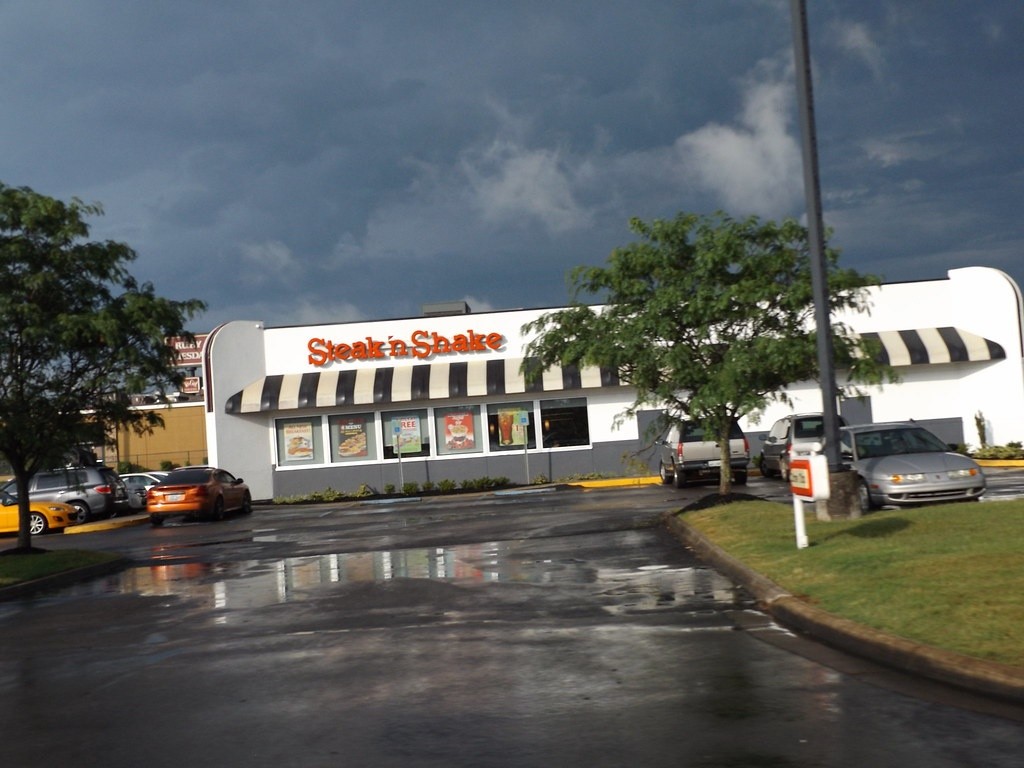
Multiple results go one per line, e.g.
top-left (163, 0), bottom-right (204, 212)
top-left (146, 466), bottom-right (252, 526)
top-left (816, 419), bottom-right (987, 513)
top-left (0, 490), bottom-right (80, 536)
top-left (116, 471), bottom-right (173, 509)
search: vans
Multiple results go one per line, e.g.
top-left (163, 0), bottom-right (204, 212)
top-left (654, 414), bottom-right (751, 490)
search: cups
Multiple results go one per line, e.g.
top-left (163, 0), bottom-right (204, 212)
top-left (498, 415), bottom-right (515, 444)
top-left (450, 425), bottom-right (467, 444)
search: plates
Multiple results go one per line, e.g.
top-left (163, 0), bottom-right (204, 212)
top-left (286, 437), bottom-right (312, 458)
top-left (339, 433), bottom-right (366, 457)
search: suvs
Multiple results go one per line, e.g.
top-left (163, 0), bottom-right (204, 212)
top-left (1, 460), bottom-right (129, 526)
top-left (758, 412), bottom-right (850, 482)
